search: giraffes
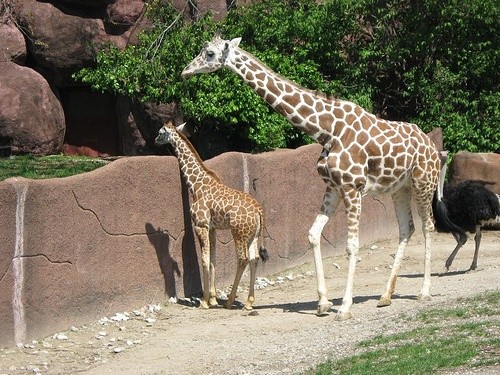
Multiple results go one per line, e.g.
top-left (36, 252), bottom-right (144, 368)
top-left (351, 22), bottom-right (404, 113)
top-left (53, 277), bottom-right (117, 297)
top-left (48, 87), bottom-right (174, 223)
top-left (182, 36), bottom-right (468, 320)
top-left (154, 121), bottom-right (269, 311)
